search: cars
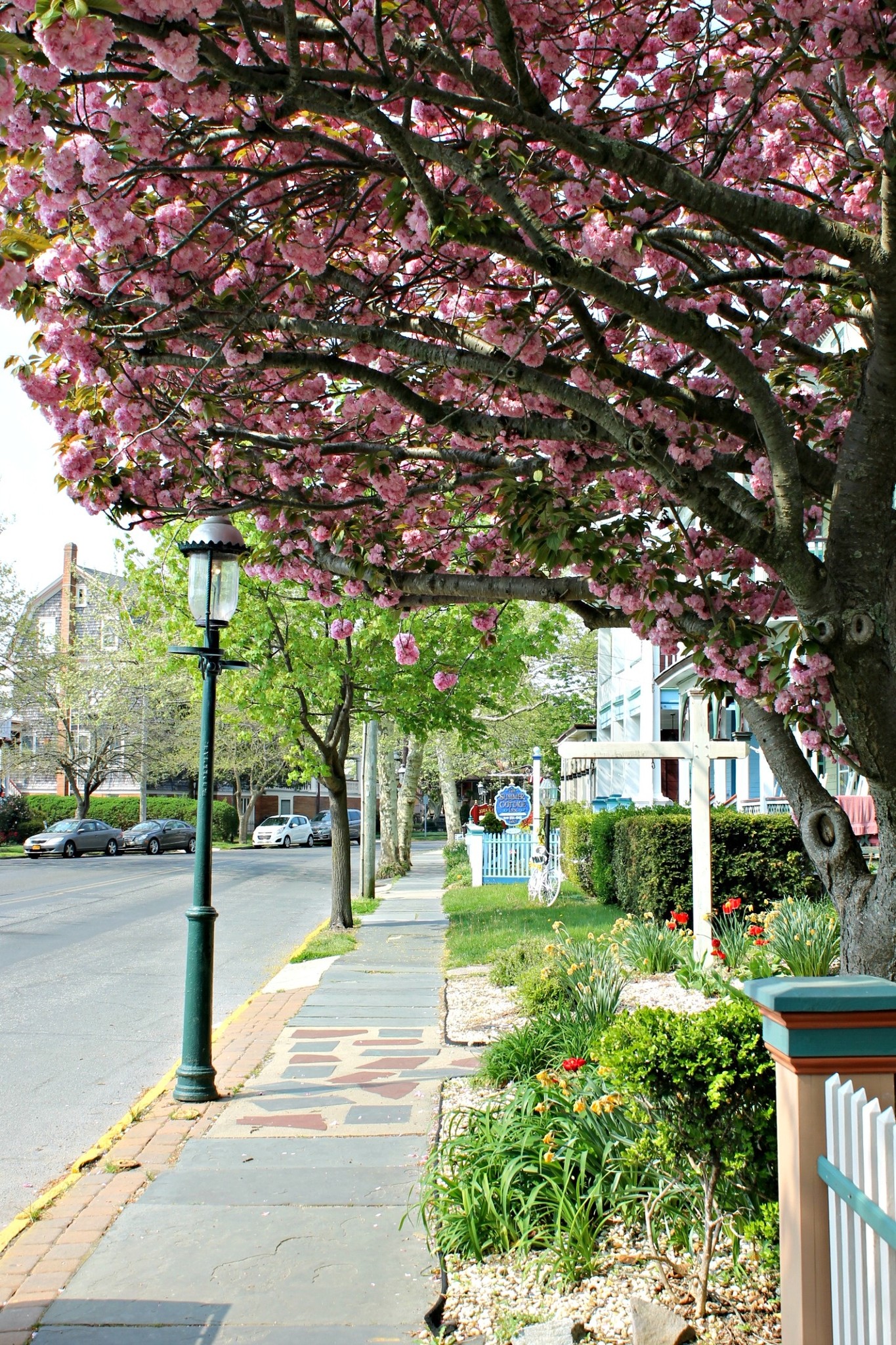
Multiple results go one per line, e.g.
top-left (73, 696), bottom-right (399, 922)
top-left (309, 808), bottom-right (361, 847)
top-left (252, 814), bottom-right (315, 849)
top-left (121, 818), bottom-right (196, 856)
top-left (23, 819), bottom-right (125, 859)
top-left (433, 813), bottom-right (447, 832)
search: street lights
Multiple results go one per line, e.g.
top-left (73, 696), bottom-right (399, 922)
top-left (171, 500), bottom-right (256, 1104)
